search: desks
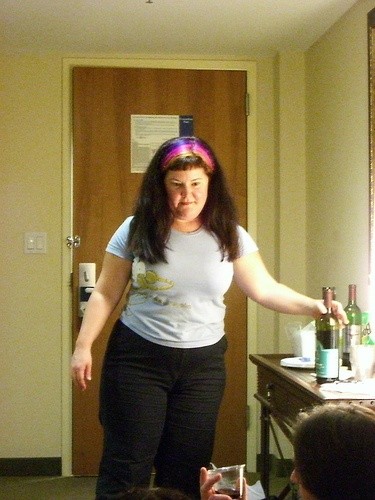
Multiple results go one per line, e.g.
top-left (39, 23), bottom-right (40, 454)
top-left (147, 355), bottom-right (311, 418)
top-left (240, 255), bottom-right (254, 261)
top-left (249, 353), bottom-right (375, 500)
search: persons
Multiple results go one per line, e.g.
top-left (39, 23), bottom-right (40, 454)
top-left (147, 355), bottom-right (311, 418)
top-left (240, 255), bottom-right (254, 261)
top-left (199, 404), bottom-right (375, 500)
top-left (70, 136), bottom-right (349, 500)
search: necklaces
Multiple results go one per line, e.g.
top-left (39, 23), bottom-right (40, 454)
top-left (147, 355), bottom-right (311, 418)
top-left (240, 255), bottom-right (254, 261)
top-left (170, 221), bottom-right (203, 234)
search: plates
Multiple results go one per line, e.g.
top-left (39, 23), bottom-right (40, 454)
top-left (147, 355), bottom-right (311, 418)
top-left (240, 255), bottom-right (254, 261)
top-left (280, 356), bottom-right (342, 369)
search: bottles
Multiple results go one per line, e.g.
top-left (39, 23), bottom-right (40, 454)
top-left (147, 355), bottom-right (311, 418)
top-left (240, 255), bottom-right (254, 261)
top-left (342, 285), bottom-right (362, 366)
top-left (315, 287), bottom-right (340, 384)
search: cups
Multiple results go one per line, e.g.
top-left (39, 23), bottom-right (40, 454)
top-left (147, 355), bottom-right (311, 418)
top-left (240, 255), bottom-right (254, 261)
top-left (208, 464), bottom-right (246, 499)
top-left (349, 345), bottom-right (374, 379)
